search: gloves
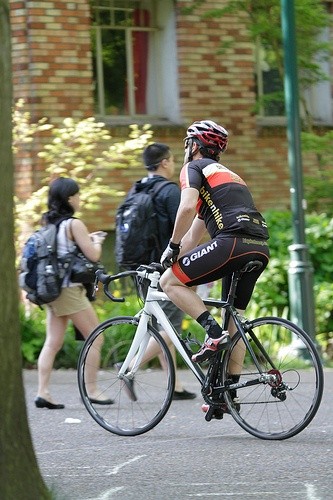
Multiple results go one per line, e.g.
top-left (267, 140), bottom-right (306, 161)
top-left (159, 240), bottom-right (180, 268)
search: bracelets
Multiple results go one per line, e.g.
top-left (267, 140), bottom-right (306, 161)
top-left (169, 237), bottom-right (182, 249)
top-left (94, 241), bottom-right (103, 245)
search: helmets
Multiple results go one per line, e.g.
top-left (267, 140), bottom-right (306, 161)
top-left (183, 118), bottom-right (229, 158)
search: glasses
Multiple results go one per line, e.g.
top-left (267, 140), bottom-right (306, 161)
top-left (184, 140), bottom-right (189, 149)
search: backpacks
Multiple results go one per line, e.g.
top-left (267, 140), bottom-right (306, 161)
top-left (115, 181), bottom-right (177, 271)
top-left (17, 215), bottom-right (78, 311)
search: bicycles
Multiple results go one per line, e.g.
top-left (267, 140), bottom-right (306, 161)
top-left (74, 258), bottom-right (325, 442)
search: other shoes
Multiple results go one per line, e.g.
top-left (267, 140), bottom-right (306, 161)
top-left (35, 396), bottom-right (66, 410)
top-left (114, 361), bottom-right (137, 401)
top-left (86, 396), bottom-right (114, 406)
top-left (170, 388), bottom-right (197, 400)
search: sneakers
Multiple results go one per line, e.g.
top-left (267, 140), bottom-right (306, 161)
top-left (201, 396), bottom-right (241, 414)
top-left (190, 330), bottom-right (233, 363)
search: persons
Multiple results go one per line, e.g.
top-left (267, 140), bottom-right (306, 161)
top-left (33, 178), bottom-right (114, 409)
top-left (162, 120), bottom-right (270, 415)
top-left (113, 143), bottom-right (198, 400)
